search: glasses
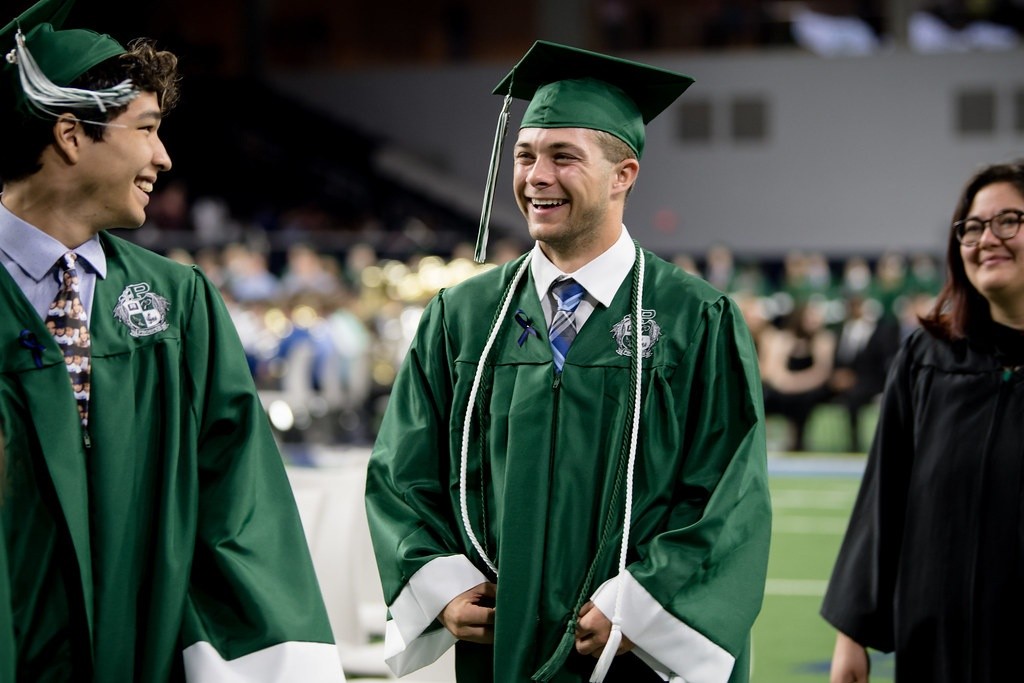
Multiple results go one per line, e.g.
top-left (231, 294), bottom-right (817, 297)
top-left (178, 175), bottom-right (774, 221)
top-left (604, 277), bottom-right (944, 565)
top-left (954, 209), bottom-right (1023, 247)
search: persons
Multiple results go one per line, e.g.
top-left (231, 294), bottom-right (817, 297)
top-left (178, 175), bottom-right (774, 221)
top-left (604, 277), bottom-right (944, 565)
top-left (366, 42), bottom-right (773, 683)
top-left (138, 189), bottom-right (966, 453)
top-left (0, 1), bottom-right (348, 683)
top-left (818, 162), bottom-right (1024, 683)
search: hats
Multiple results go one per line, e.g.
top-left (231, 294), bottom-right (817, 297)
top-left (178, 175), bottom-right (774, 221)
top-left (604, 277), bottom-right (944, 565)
top-left (473, 39), bottom-right (695, 263)
top-left (0, 0), bottom-right (131, 125)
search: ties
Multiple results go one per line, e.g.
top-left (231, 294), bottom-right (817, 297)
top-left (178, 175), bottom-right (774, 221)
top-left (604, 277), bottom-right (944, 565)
top-left (45, 251), bottom-right (91, 448)
top-left (549, 281), bottom-right (585, 374)
